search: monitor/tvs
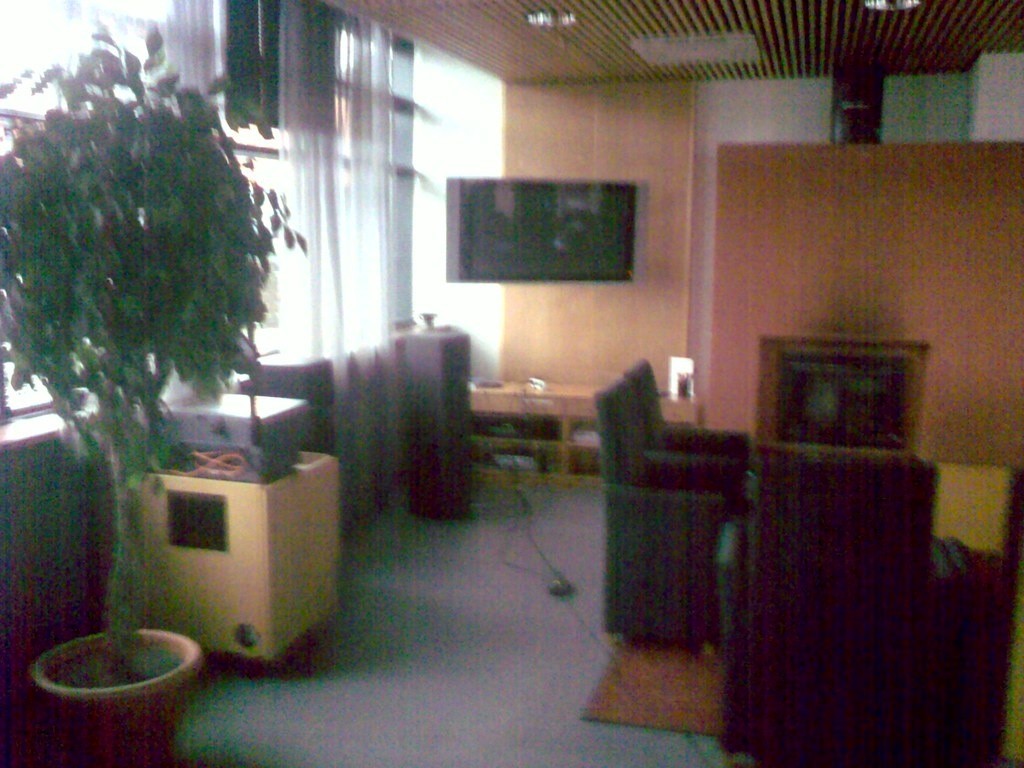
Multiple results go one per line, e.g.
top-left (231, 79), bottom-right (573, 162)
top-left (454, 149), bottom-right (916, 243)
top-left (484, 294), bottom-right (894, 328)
top-left (444, 178), bottom-right (652, 284)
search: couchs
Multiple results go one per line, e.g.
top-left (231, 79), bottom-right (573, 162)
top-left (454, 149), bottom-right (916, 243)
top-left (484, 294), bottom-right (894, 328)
top-left (623, 358), bottom-right (749, 522)
top-left (716, 442), bottom-right (971, 768)
top-left (594, 377), bottom-right (744, 651)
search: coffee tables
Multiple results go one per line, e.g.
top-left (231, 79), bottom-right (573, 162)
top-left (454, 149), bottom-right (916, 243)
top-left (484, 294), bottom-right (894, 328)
top-left (931, 463), bottom-right (1012, 606)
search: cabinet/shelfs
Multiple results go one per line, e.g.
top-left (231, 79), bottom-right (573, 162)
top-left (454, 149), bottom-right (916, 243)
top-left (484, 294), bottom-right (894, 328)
top-left (470, 393), bottom-right (697, 489)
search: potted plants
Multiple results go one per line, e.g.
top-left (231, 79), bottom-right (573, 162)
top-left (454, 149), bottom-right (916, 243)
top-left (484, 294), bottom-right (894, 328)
top-left (0, 23), bottom-right (308, 768)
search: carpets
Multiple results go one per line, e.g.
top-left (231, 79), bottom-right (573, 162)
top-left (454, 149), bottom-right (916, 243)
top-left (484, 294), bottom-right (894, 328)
top-left (578, 469), bottom-right (1024, 762)
